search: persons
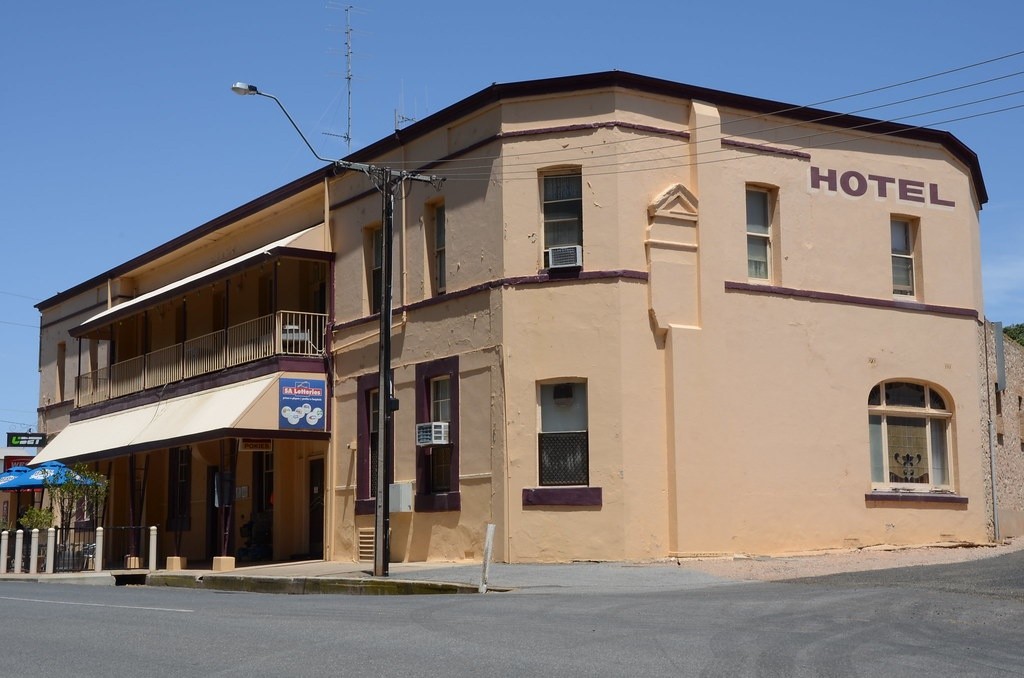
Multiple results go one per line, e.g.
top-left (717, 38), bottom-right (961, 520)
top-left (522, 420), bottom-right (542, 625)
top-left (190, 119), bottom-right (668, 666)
top-left (15, 518), bottom-right (30, 573)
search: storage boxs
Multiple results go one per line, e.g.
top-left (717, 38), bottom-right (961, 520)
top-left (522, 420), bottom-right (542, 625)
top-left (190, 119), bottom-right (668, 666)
top-left (87, 557), bottom-right (95, 570)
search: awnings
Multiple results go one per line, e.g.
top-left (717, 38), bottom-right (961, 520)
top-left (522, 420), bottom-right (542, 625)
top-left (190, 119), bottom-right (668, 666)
top-left (26, 371), bottom-right (332, 467)
top-left (69, 223), bottom-right (331, 339)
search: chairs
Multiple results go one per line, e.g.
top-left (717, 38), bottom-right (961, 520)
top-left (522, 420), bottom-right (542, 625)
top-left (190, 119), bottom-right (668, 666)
top-left (82, 543), bottom-right (96, 570)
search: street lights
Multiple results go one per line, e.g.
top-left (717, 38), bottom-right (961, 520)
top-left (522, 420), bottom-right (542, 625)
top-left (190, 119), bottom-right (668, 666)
top-left (232, 81), bottom-right (449, 577)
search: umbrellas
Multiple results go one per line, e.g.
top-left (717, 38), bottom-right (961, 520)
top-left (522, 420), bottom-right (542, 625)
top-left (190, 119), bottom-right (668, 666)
top-left (0, 467), bottom-right (32, 485)
top-left (0, 460), bottom-right (102, 488)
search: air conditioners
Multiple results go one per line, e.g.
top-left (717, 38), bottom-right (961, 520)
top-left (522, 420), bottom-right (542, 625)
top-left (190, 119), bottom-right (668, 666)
top-left (416, 422), bottom-right (450, 447)
top-left (548, 245), bottom-right (582, 269)
